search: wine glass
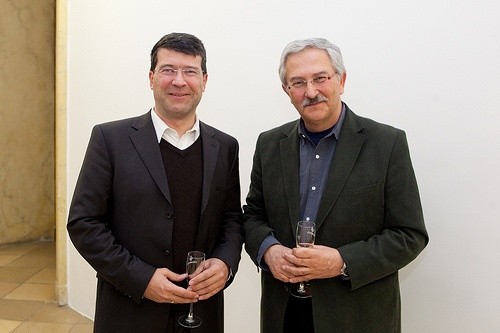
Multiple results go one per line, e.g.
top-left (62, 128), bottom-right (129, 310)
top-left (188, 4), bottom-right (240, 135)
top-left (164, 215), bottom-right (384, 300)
top-left (178, 251), bottom-right (205, 327)
top-left (290, 221), bottom-right (316, 298)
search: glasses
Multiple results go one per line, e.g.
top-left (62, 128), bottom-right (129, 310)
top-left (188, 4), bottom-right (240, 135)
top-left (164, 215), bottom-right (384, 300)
top-left (153, 67), bottom-right (204, 77)
top-left (284, 71), bottom-right (337, 92)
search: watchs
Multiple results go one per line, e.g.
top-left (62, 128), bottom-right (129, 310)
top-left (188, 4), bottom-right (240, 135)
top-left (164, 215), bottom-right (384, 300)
top-left (340, 263), bottom-right (349, 276)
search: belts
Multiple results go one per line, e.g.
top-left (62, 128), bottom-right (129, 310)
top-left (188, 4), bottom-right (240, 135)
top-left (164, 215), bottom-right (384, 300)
top-left (289, 283), bottom-right (313, 298)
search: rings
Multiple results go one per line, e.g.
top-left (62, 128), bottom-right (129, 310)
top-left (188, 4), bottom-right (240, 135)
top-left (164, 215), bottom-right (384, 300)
top-left (171, 301), bottom-right (174, 303)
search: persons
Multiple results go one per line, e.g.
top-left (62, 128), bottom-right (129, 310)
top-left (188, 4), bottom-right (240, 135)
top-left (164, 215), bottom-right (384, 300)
top-left (242, 38), bottom-right (429, 333)
top-left (66, 32), bottom-right (243, 333)
